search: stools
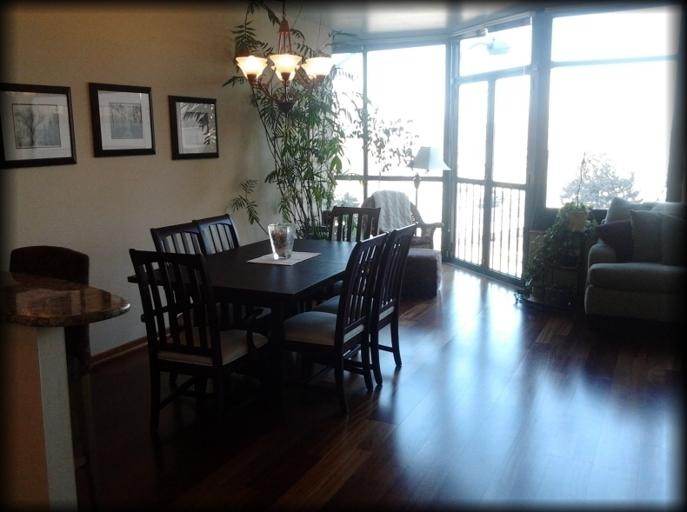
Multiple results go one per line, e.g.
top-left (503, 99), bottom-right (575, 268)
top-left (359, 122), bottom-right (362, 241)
top-left (403, 248), bottom-right (441, 297)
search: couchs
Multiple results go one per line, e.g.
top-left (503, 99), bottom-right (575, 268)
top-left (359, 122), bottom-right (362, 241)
top-left (583, 196), bottom-right (686, 323)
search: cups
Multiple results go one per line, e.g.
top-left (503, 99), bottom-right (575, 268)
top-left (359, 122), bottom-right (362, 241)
top-left (268, 223), bottom-right (297, 260)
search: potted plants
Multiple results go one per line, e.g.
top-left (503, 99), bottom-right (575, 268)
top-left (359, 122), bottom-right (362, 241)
top-left (523, 227), bottom-right (552, 298)
top-left (545, 195), bottom-right (590, 256)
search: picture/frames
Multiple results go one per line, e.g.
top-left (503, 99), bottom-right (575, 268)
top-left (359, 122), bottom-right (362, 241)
top-left (0, 84), bottom-right (77, 168)
top-left (168, 95), bottom-right (219, 160)
top-left (88, 82), bottom-right (156, 157)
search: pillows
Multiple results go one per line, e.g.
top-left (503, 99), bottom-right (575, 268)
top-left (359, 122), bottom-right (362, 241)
top-left (603, 219), bottom-right (632, 262)
top-left (659, 211), bottom-right (686, 265)
top-left (627, 208), bottom-right (659, 261)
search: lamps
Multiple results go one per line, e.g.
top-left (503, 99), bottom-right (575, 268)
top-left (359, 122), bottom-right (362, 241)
top-left (407, 146), bottom-right (453, 207)
top-left (235, 0), bottom-right (337, 114)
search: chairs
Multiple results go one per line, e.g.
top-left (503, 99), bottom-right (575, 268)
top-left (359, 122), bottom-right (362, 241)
top-left (359, 190), bottom-right (444, 249)
top-left (9, 246), bottom-right (100, 484)
